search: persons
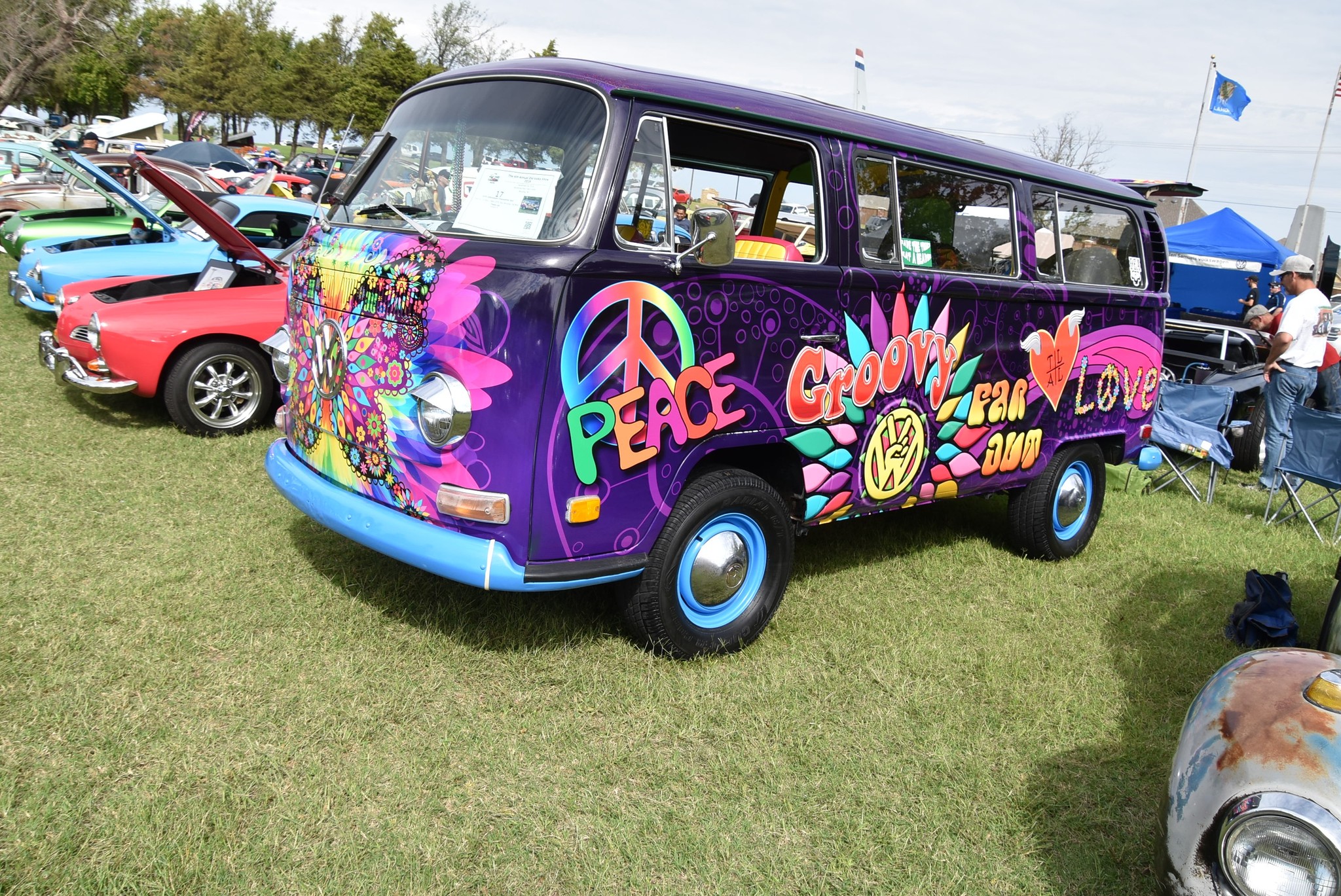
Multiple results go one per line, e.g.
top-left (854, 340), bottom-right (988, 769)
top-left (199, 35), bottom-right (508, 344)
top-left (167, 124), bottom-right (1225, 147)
top-left (409, 171), bottom-right (432, 213)
top-left (664, 205), bottom-right (691, 235)
top-left (1264, 281), bottom-right (1287, 317)
top-left (74, 132), bottom-right (116, 192)
top-left (673, 204), bottom-right (682, 218)
top-left (1237, 275), bottom-right (1260, 321)
top-left (424, 170), bottom-right (443, 215)
top-left (1242, 304), bottom-right (1341, 414)
top-left (1237, 254), bottom-right (1334, 495)
top-left (134, 143), bottom-right (146, 155)
top-left (432, 169), bottom-right (451, 215)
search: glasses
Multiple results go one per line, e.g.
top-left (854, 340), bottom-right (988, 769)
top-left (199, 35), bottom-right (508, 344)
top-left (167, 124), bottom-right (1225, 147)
top-left (1278, 272), bottom-right (1290, 281)
top-left (441, 175), bottom-right (452, 179)
top-left (1248, 317), bottom-right (1258, 325)
top-left (1270, 286), bottom-right (1279, 288)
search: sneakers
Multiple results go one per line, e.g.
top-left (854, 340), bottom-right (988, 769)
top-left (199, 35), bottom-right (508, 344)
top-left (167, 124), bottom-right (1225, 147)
top-left (1238, 482), bottom-right (1269, 495)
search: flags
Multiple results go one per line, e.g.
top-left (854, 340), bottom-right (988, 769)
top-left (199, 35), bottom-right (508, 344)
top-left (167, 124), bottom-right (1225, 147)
top-left (855, 48), bottom-right (865, 71)
top-left (1209, 71), bottom-right (1252, 122)
top-left (1334, 66), bottom-right (1341, 96)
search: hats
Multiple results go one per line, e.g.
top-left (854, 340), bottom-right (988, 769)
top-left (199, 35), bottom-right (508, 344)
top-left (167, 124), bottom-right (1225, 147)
top-left (135, 143), bottom-right (146, 150)
top-left (1268, 281), bottom-right (1282, 287)
top-left (1269, 254), bottom-right (1315, 276)
top-left (1243, 304), bottom-right (1269, 325)
top-left (438, 169), bottom-right (453, 177)
top-left (84, 132), bottom-right (102, 142)
top-left (300, 187), bottom-right (313, 195)
top-left (1245, 275), bottom-right (1259, 281)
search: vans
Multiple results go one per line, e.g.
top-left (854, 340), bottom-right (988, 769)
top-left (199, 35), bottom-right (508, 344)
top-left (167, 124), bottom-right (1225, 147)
top-left (265, 56), bottom-right (1209, 665)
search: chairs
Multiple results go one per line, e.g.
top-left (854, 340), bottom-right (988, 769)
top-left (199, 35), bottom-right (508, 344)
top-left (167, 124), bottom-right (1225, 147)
top-left (263, 219), bottom-right (291, 250)
top-left (734, 234), bottom-right (805, 264)
top-left (1140, 379), bottom-right (1235, 507)
top-left (1262, 402), bottom-right (1341, 548)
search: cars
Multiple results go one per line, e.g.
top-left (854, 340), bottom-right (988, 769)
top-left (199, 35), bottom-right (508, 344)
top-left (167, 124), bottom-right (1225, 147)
top-left (0, 187), bottom-right (277, 262)
top-left (8, 153), bottom-right (341, 329)
top-left (1, 150), bottom-right (236, 222)
top-left (38, 152), bottom-right (298, 440)
top-left (1, 113), bottom-right (888, 287)
top-left (1151, 556), bottom-right (1340, 896)
